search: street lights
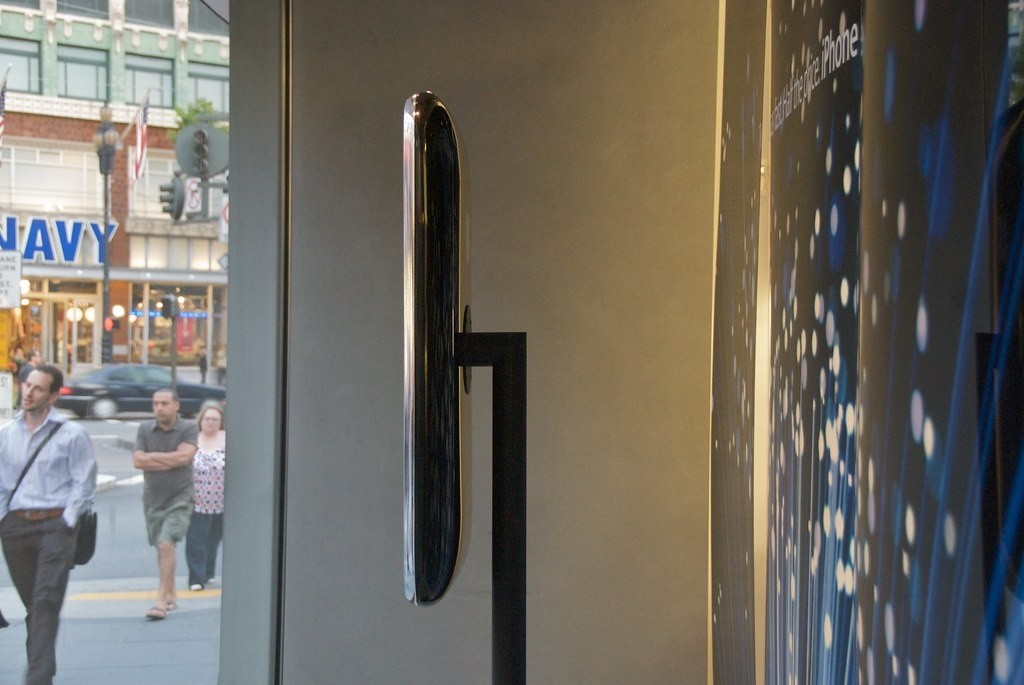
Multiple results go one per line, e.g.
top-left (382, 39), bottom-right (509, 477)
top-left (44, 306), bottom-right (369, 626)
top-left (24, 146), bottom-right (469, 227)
top-left (94, 102), bottom-right (119, 360)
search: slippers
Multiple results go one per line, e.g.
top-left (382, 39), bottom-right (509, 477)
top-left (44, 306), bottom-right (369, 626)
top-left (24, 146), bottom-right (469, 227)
top-left (146, 607), bottom-right (166, 618)
top-left (166, 601), bottom-right (177, 610)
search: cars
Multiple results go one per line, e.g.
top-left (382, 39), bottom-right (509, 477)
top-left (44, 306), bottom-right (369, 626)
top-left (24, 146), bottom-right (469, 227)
top-left (54, 364), bottom-right (225, 419)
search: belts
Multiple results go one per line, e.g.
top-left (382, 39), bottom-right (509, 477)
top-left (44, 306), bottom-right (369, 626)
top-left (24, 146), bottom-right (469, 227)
top-left (13, 509), bottom-right (63, 521)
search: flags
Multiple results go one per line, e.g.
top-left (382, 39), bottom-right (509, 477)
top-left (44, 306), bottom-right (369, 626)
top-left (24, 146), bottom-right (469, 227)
top-left (135, 100), bottom-right (148, 179)
top-left (0, 77), bottom-right (5, 167)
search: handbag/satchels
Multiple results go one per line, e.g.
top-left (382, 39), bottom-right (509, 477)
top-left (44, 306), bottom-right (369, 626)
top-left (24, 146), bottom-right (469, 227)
top-left (74, 510), bottom-right (97, 564)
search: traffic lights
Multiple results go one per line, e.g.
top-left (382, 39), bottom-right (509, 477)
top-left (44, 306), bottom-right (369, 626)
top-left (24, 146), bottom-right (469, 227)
top-left (161, 295), bottom-right (176, 319)
top-left (103, 317), bottom-right (118, 332)
top-left (160, 176), bottom-right (187, 221)
top-left (175, 123), bottom-right (228, 181)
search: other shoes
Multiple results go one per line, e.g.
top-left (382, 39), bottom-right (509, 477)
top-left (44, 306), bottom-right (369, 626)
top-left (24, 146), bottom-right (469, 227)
top-left (208, 579), bottom-right (215, 583)
top-left (190, 583), bottom-right (201, 590)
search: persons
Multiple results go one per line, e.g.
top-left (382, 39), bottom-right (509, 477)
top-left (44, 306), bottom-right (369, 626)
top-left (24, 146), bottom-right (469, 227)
top-left (132, 388), bottom-right (198, 619)
top-left (15, 351), bottom-right (40, 413)
top-left (186, 404), bottom-right (225, 590)
top-left (1, 367), bottom-right (96, 685)
